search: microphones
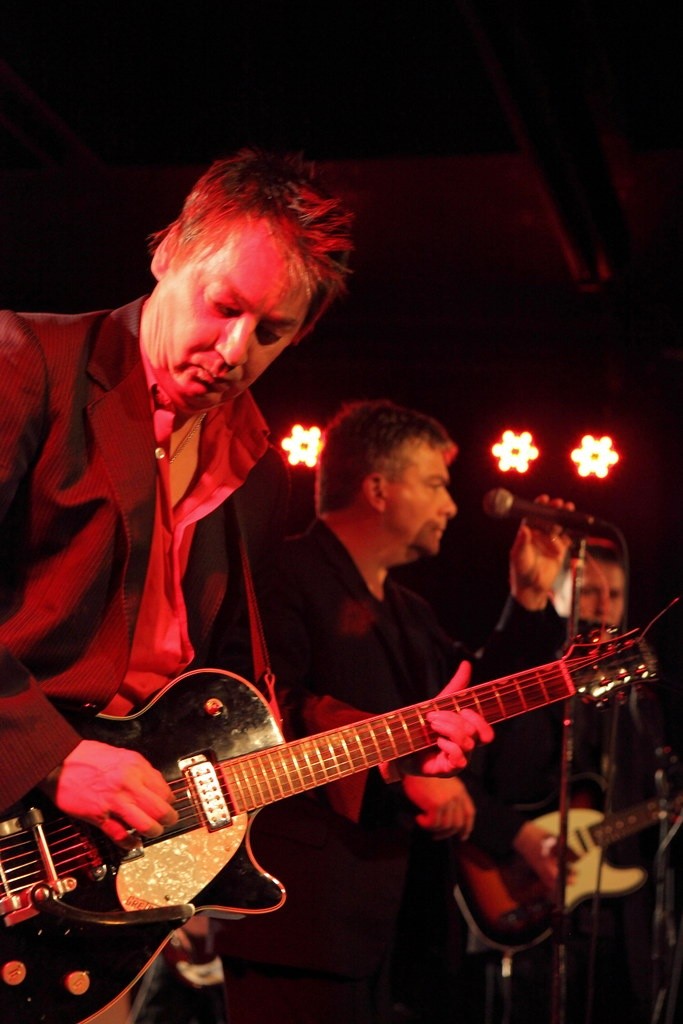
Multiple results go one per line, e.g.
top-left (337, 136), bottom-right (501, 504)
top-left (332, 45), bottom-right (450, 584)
top-left (483, 488), bottom-right (602, 527)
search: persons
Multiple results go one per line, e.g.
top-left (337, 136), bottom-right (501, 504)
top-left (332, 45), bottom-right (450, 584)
top-left (208, 401), bottom-right (576, 1024)
top-left (503, 540), bottom-right (683, 1024)
top-left (0, 149), bottom-right (495, 1024)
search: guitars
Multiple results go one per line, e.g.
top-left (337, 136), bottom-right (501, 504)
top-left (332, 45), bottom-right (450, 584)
top-left (0, 592), bottom-right (683, 1023)
top-left (436, 771), bottom-right (682, 956)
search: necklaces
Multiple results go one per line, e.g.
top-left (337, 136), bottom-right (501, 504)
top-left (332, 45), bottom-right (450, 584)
top-left (170, 412), bottom-right (206, 468)
